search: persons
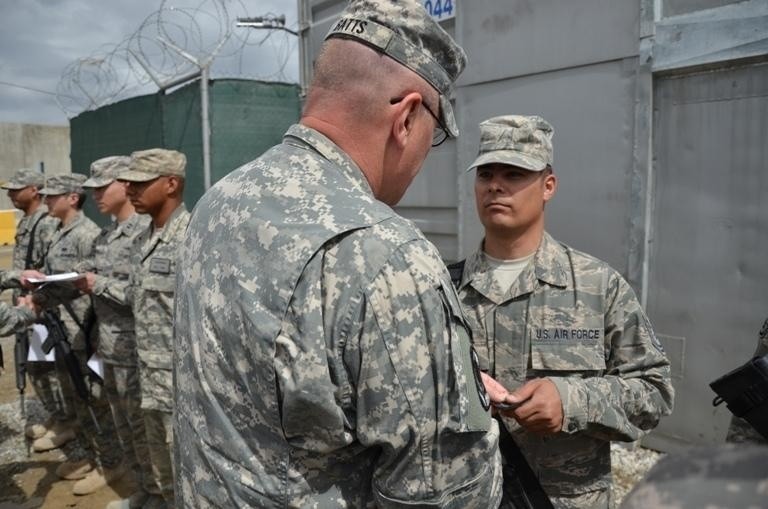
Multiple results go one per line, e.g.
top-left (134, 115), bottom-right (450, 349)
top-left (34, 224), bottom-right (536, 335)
top-left (0, 168), bottom-right (77, 452)
top-left (0, 268), bottom-right (46, 337)
top-left (172, 0), bottom-right (503, 508)
top-left (36, 173), bottom-right (126, 495)
top-left (106, 147), bottom-right (192, 508)
top-left (25, 155), bottom-right (165, 509)
top-left (447, 115), bottom-right (676, 509)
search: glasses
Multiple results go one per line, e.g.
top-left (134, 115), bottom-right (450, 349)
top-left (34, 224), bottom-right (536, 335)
top-left (390, 97), bottom-right (448, 146)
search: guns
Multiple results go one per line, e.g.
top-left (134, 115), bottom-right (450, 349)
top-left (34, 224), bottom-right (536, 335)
top-left (41, 305), bottom-right (101, 439)
top-left (16, 331), bottom-right (27, 419)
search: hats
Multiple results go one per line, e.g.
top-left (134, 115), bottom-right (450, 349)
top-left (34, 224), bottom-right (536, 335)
top-left (1, 148), bottom-right (186, 195)
top-left (325, 0), bottom-right (468, 137)
top-left (467, 115), bottom-right (554, 172)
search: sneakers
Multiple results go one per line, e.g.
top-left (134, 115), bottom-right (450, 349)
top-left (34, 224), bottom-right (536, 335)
top-left (25, 419), bottom-right (74, 452)
top-left (107, 489), bottom-right (167, 509)
top-left (56, 460), bottom-right (127, 494)
